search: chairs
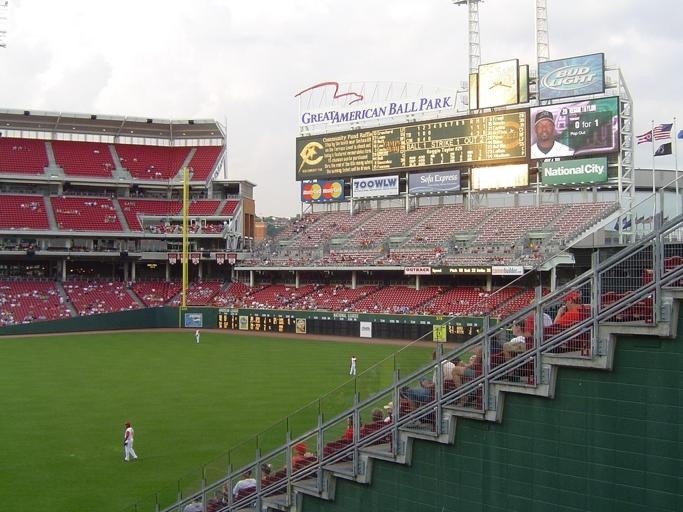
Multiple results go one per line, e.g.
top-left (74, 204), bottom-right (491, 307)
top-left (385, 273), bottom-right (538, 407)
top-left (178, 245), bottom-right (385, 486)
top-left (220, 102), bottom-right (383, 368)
top-left (318, 353), bottom-right (484, 459)
top-left (1, 139), bottom-right (223, 190)
top-left (486, 254), bottom-right (678, 390)
top-left (0, 275), bottom-right (240, 329)
top-left (205, 451), bottom-right (318, 508)
top-left (1, 193), bottom-right (241, 238)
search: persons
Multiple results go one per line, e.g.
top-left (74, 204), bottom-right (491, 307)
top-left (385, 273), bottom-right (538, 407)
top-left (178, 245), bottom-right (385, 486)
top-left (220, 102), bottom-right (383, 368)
top-left (94, 150), bottom-right (162, 177)
top-left (242, 212), bottom-right (543, 319)
top-left (194, 327), bottom-right (201, 344)
top-left (20, 194), bottom-right (183, 233)
top-left (178, 442), bottom-right (316, 511)
top-left (0, 240), bottom-right (181, 325)
top-left (188, 280), bottom-right (242, 309)
top-left (338, 289), bottom-right (592, 441)
top-left (122, 422), bottom-right (138, 461)
top-left (530, 110), bottom-right (574, 158)
top-left (191, 221), bottom-right (228, 233)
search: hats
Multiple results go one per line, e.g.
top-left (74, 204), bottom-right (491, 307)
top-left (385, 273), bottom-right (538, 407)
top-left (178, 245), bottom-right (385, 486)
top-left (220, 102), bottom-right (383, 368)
top-left (295, 445), bottom-right (306, 452)
top-left (125, 423), bottom-right (130, 425)
top-left (383, 402), bottom-right (393, 409)
top-left (562, 292), bottom-right (581, 301)
top-left (535, 111), bottom-right (554, 125)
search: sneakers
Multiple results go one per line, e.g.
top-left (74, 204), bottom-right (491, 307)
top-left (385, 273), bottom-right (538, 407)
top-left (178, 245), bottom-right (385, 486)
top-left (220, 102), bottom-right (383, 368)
top-left (406, 420), bottom-right (421, 428)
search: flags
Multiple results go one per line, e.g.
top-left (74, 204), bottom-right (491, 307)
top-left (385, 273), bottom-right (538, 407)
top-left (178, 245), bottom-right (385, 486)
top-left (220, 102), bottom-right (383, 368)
top-left (652, 142), bottom-right (672, 156)
top-left (652, 123), bottom-right (672, 141)
top-left (635, 130), bottom-right (652, 144)
top-left (676, 129), bottom-right (682, 140)
